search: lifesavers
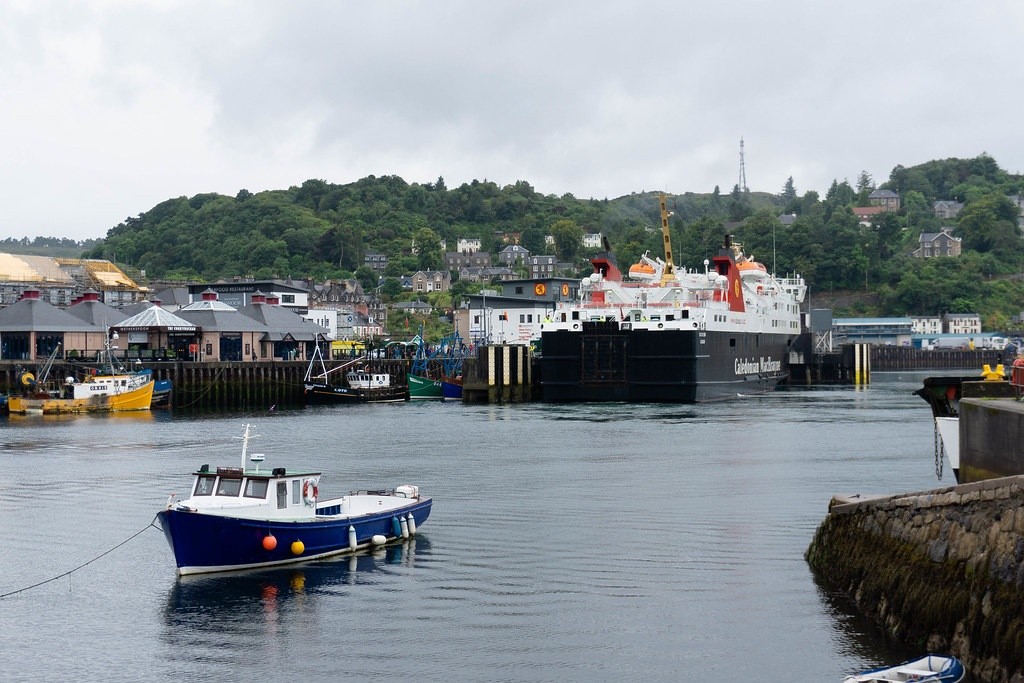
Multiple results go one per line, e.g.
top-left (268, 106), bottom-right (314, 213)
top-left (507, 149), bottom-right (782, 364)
top-left (303, 478), bottom-right (318, 505)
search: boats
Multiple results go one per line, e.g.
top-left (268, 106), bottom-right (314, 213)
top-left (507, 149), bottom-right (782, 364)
top-left (536, 232), bottom-right (809, 407)
top-left (405, 372), bottom-right (442, 401)
top-left (301, 332), bottom-right (409, 405)
top-left (6, 341), bottom-right (156, 414)
top-left (156, 424), bottom-right (433, 584)
top-left (842, 652), bottom-right (969, 683)
top-left (440, 370), bottom-right (463, 402)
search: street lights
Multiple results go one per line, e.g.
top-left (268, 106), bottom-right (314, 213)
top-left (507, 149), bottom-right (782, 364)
top-left (482, 278), bottom-right (490, 346)
